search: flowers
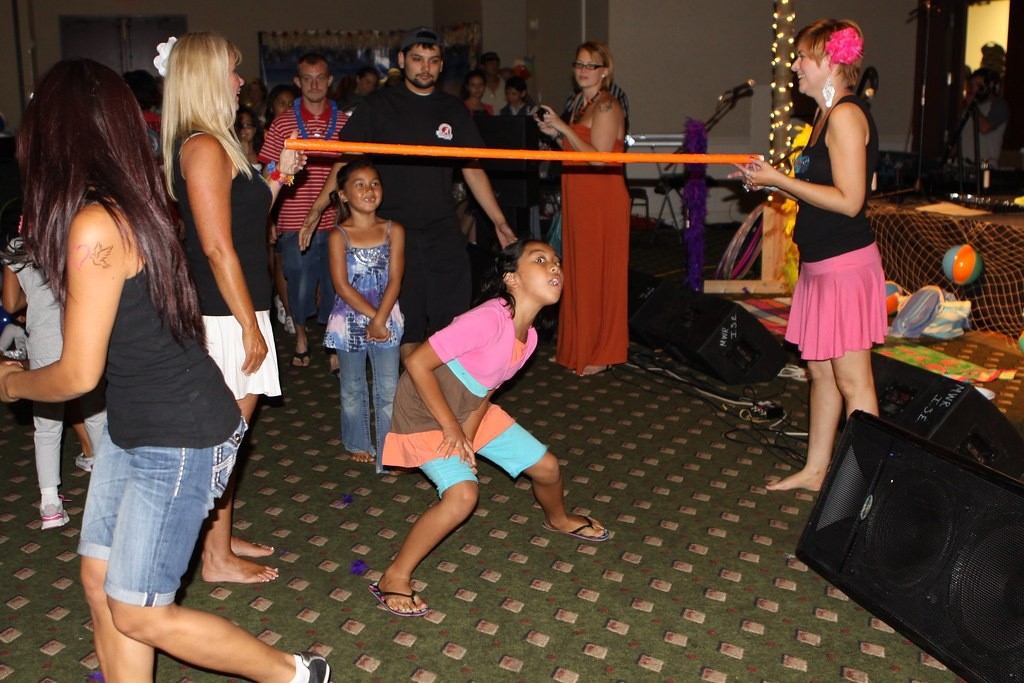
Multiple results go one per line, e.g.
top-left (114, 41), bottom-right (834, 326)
top-left (153, 36), bottom-right (177, 75)
top-left (824, 27), bottom-right (864, 63)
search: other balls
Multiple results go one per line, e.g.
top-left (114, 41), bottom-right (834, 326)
top-left (942, 244), bottom-right (982, 286)
top-left (885, 279), bottom-right (902, 315)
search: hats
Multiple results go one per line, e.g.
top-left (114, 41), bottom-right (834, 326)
top-left (401, 27), bottom-right (442, 51)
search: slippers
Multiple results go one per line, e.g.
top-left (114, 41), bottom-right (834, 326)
top-left (543, 515), bottom-right (610, 541)
top-left (289, 346), bottom-right (310, 369)
top-left (329, 352), bottom-right (340, 378)
top-left (368, 581), bottom-right (428, 617)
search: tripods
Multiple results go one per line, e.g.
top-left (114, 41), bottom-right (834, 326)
top-left (868, 0), bottom-right (937, 204)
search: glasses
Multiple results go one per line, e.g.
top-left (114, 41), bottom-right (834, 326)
top-left (572, 61), bottom-right (605, 70)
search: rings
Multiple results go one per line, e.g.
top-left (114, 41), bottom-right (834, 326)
top-left (746, 170), bottom-right (750, 176)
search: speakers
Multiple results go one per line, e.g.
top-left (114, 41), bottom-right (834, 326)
top-left (836, 351), bottom-right (1024, 480)
top-left (472, 207), bottom-right (531, 258)
top-left (475, 114), bottom-right (540, 209)
top-left (654, 287), bottom-right (791, 385)
top-left (626, 268), bottom-right (685, 349)
top-left (795, 410), bottom-right (1024, 683)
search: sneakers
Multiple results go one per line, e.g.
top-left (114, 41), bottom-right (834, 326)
top-left (295, 651), bottom-right (331, 683)
top-left (284, 316), bottom-right (296, 334)
top-left (275, 295), bottom-right (286, 325)
top-left (39, 495), bottom-right (72, 530)
top-left (75, 451), bottom-right (95, 471)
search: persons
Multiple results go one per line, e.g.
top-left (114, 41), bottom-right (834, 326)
top-left (322, 160), bottom-right (405, 476)
top-left (534, 41), bottom-right (630, 375)
top-left (234, 52), bottom-right (380, 377)
top-left (0, 31), bottom-right (281, 585)
top-left (298, 27), bottom-right (517, 378)
top-left (950, 68), bottom-right (1009, 196)
top-left (441, 52), bottom-right (538, 244)
top-left (0, 59), bottom-right (332, 683)
top-left (368, 239), bottom-right (608, 617)
top-left (733, 17), bottom-right (888, 490)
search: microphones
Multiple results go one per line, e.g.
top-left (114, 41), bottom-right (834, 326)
top-left (724, 80), bottom-right (756, 95)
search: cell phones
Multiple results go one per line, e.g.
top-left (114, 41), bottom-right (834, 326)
top-left (537, 107), bottom-right (549, 122)
